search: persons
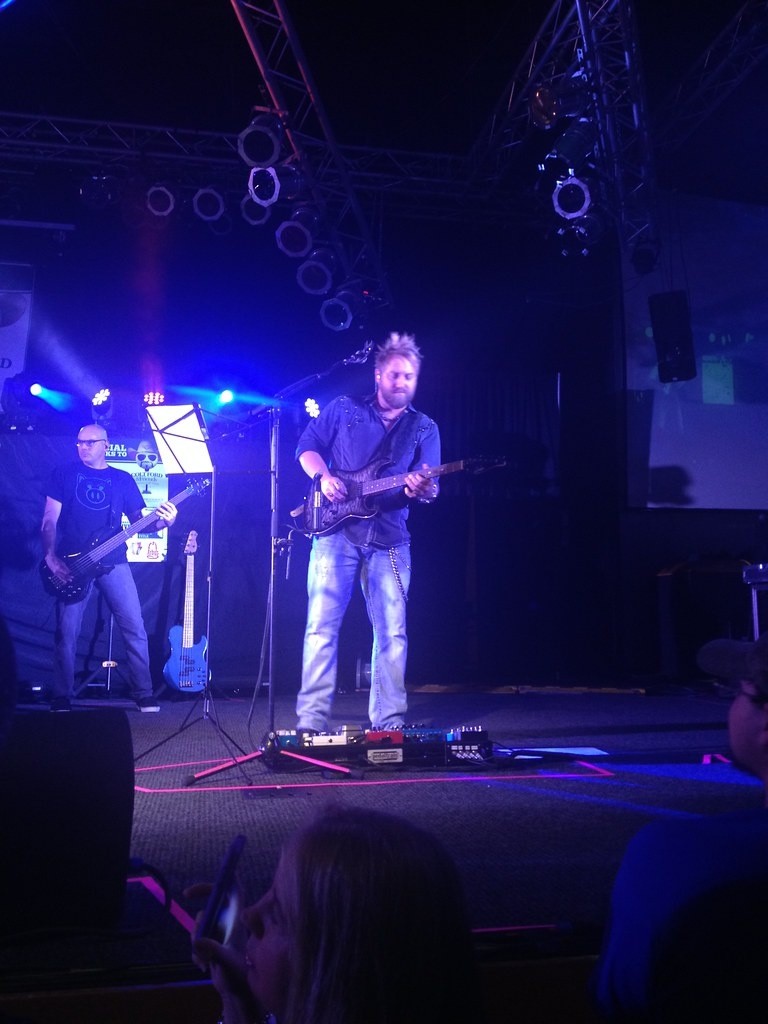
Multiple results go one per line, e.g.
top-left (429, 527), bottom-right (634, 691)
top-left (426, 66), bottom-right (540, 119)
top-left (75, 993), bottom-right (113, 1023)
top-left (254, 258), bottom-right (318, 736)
top-left (586, 643), bottom-right (768, 1024)
top-left (293, 332), bottom-right (441, 764)
top-left (41, 425), bottom-right (179, 713)
top-left (188, 805), bottom-right (491, 1024)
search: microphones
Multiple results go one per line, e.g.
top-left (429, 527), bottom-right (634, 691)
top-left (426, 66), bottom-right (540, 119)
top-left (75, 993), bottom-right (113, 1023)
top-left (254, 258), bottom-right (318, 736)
top-left (360, 341), bottom-right (372, 364)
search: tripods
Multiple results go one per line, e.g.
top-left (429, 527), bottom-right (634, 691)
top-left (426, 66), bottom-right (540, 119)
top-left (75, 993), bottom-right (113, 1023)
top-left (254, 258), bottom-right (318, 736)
top-left (71, 613), bottom-right (130, 699)
top-left (135, 350), bottom-right (366, 787)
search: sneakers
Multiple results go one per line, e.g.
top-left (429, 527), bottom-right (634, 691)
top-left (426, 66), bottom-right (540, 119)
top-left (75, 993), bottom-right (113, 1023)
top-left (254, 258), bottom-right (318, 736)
top-left (49, 695), bottom-right (71, 712)
top-left (136, 696), bottom-right (160, 713)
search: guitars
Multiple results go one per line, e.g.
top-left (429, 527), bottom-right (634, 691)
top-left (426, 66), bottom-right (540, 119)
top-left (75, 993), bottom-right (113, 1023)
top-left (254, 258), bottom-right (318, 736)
top-left (294, 449), bottom-right (519, 538)
top-left (37, 473), bottom-right (214, 607)
top-left (159, 527), bottom-right (212, 696)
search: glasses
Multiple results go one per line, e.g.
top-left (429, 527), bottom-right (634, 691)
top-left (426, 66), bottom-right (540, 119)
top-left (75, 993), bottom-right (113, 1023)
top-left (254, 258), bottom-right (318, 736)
top-left (713, 677), bottom-right (763, 702)
top-left (75, 439), bottom-right (106, 448)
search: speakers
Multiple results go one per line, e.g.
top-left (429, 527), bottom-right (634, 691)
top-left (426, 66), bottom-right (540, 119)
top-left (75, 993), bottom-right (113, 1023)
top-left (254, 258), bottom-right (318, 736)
top-left (0, 704), bottom-right (135, 937)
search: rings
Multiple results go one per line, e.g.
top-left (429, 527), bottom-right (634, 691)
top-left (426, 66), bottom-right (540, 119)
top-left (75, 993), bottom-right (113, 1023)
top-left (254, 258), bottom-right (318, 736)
top-left (329, 494), bottom-right (333, 497)
